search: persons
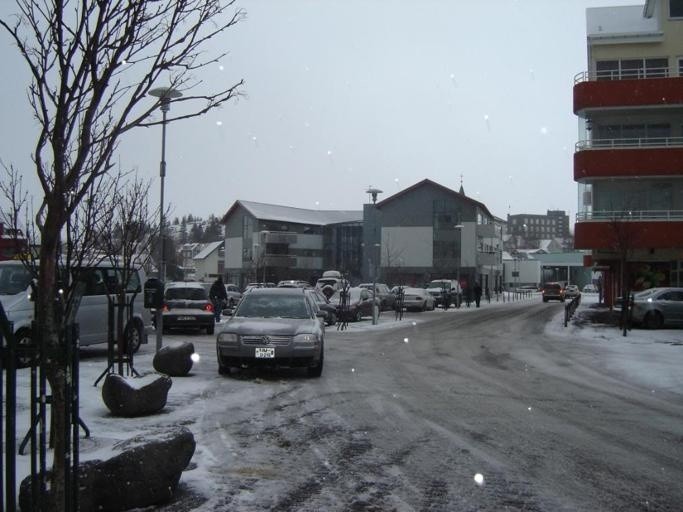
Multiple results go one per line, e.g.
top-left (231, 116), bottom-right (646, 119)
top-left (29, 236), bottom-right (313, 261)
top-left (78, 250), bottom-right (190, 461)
top-left (473, 280), bottom-right (483, 308)
top-left (208, 276), bottom-right (226, 322)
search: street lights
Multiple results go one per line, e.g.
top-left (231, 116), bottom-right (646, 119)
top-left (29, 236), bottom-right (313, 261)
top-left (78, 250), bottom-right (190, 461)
top-left (147, 84), bottom-right (182, 289)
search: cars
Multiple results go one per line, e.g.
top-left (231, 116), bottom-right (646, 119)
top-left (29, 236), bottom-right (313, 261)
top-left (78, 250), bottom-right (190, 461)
top-left (162, 282), bottom-right (218, 335)
top-left (390, 286), bottom-right (409, 299)
top-left (224, 283), bottom-right (243, 308)
top-left (542, 280), bottom-right (597, 302)
top-left (400, 287), bottom-right (436, 312)
top-left (215, 287), bottom-right (336, 378)
top-left (358, 283), bottom-right (399, 312)
top-left (611, 287), bottom-right (682, 328)
top-left (244, 270), bottom-right (352, 302)
top-left (328, 287), bottom-right (383, 322)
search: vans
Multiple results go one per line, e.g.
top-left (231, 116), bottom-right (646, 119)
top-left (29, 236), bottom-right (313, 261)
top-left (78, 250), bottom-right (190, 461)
top-left (0, 257), bottom-right (150, 366)
top-left (426, 279), bottom-right (464, 309)
